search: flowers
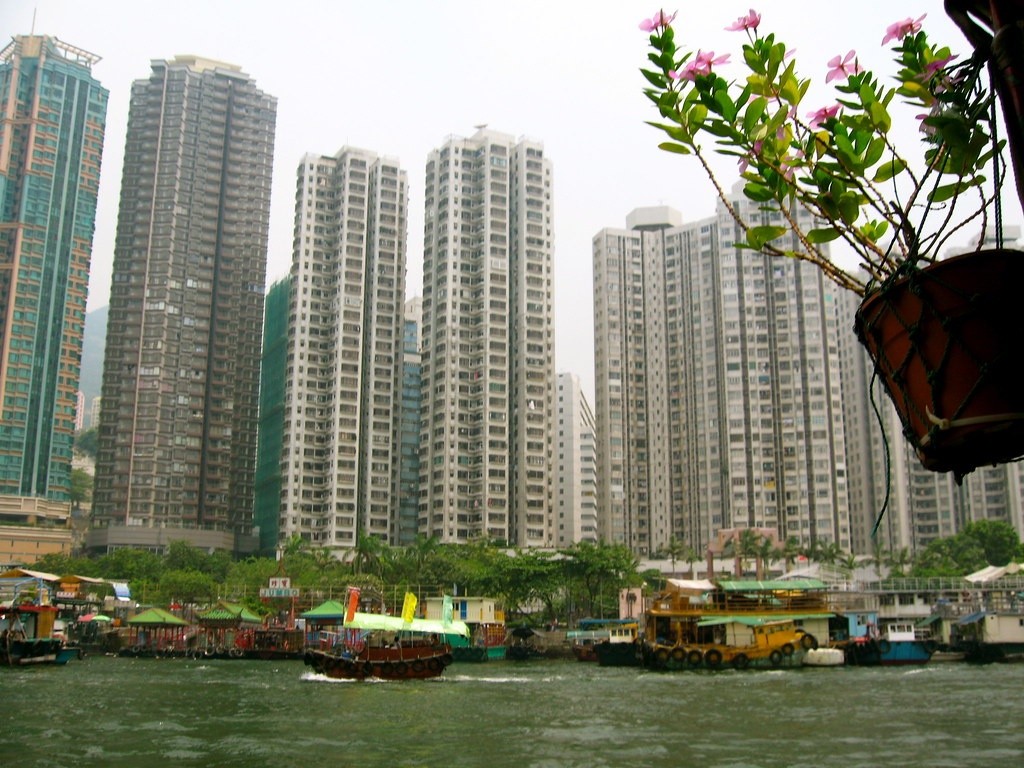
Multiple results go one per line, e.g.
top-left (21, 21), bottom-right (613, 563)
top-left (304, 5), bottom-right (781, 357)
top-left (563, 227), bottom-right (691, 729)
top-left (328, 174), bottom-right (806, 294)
top-left (640, 9), bottom-right (1007, 300)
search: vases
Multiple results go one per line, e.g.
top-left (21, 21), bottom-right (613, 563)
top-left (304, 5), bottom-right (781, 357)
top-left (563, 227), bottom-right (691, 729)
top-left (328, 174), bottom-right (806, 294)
top-left (853, 248), bottom-right (1022, 482)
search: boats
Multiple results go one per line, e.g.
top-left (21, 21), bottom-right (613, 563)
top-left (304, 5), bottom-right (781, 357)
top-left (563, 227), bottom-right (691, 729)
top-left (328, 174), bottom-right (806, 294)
top-left (0, 572), bottom-right (1024, 681)
